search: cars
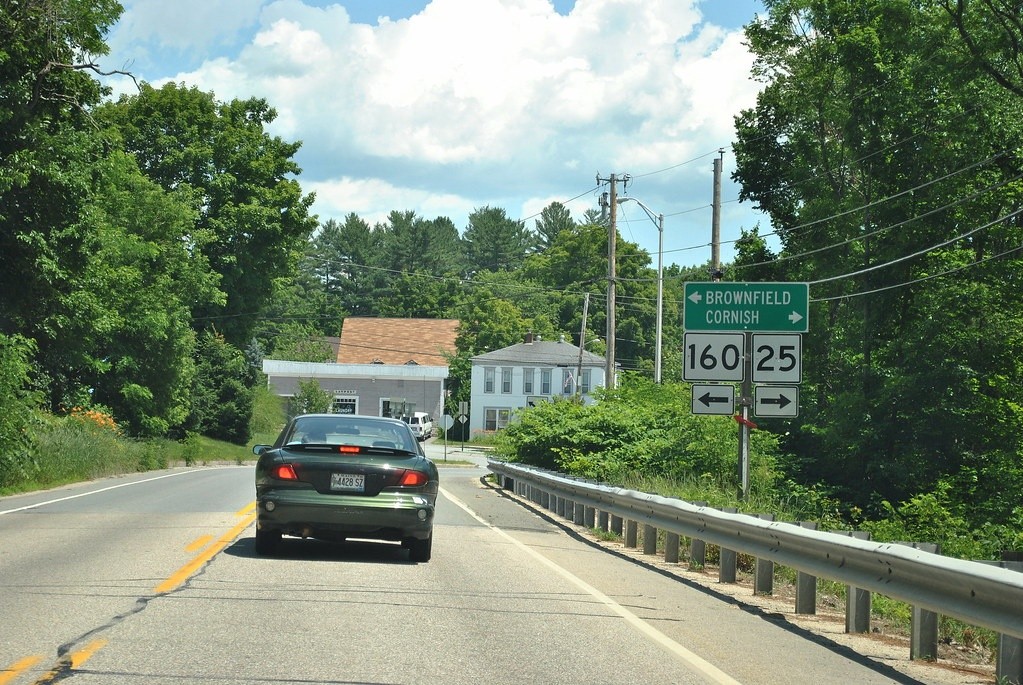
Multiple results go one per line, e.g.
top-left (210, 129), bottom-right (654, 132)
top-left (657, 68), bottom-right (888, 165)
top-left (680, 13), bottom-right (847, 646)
top-left (252, 414), bottom-right (439, 562)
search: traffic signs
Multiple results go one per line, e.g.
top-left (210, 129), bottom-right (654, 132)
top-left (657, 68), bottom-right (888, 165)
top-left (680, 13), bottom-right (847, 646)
top-left (684, 281), bottom-right (808, 334)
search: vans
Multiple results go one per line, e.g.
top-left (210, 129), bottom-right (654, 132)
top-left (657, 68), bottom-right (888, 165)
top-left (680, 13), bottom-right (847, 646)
top-left (400, 411), bottom-right (433, 441)
